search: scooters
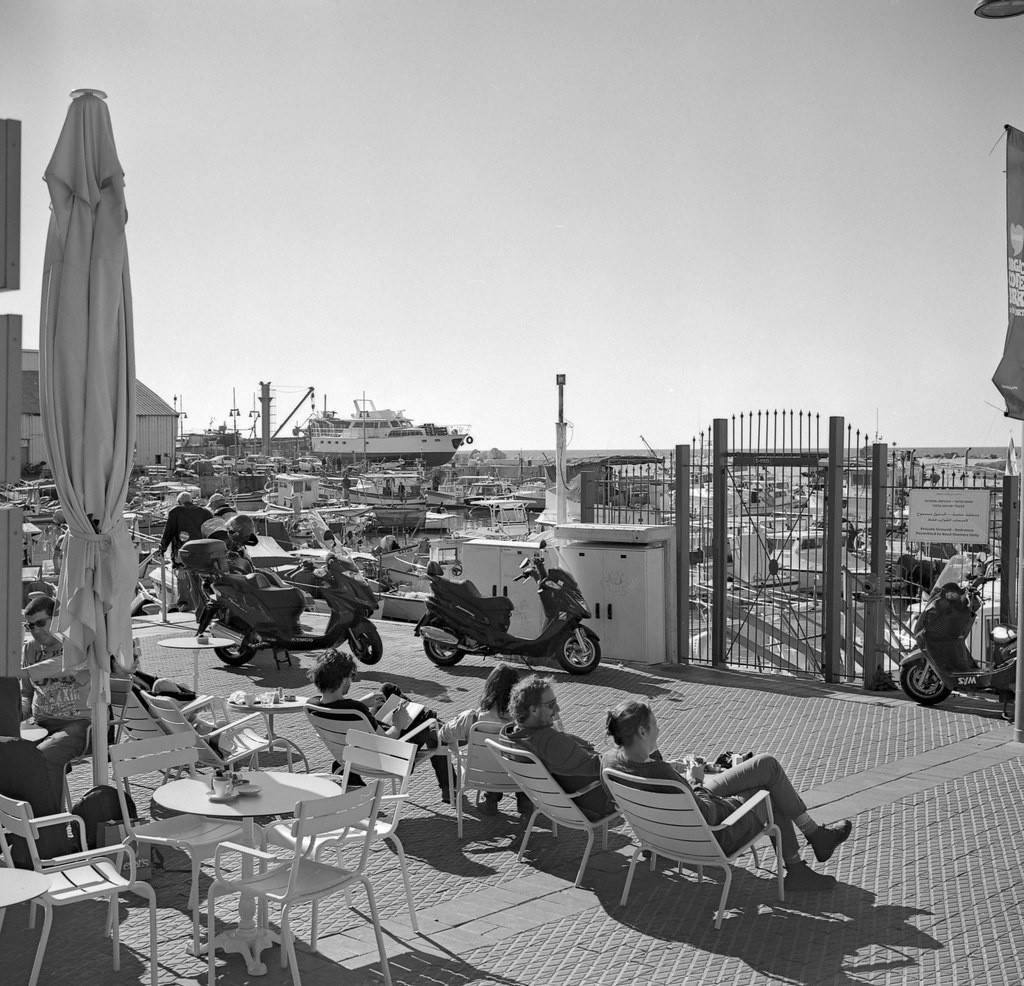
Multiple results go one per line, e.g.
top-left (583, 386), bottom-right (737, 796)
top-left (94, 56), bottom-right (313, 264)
top-left (899, 552), bottom-right (1017, 722)
top-left (178, 510), bottom-right (383, 671)
top-left (413, 539), bottom-right (601, 675)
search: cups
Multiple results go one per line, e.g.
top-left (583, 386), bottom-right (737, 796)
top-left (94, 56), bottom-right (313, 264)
top-left (213, 777), bottom-right (233, 795)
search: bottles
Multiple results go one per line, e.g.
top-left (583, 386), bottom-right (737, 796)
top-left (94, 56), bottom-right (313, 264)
top-left (274, 688), bottom-right (280, 703)
top-left (278, 687), bottom-right (283, 700)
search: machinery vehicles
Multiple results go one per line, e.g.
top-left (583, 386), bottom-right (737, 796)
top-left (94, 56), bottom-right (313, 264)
top-left (270, 386), bottom-right (315, 463)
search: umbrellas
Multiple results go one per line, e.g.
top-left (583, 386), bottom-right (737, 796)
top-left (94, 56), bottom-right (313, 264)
top-left (37, 90), bottom-right (140, 793)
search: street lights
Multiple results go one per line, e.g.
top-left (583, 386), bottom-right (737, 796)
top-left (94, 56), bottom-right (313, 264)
top-left (965, 447), bottom-right (972, 474)
top-left (555, 373), bottom-right (568, 524)
top-left (249, 392), bottom-right (260, 455)
top-left (229, 387), bottom-right (241, 471)
top-left (177, 394), bottom-right (187, 451)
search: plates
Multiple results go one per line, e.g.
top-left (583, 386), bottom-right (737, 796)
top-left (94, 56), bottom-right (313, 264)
top-left (234, 785), bottom-right (262, 796)
top-left (206, 790), bottom-right (239, 801)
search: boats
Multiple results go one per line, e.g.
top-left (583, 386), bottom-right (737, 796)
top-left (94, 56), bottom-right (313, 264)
top-left (318, 469), bottom-right (429, 530)
top-left (462, 481), bottom-right (515, 506)
top-left (310, 399), bottom-right (472, 466)
top-left (742, 485), bottom-right (798, 515)
top-left (262, 469), bottom-right (374, 547)
top-left (514, 481), bottom-right (547, 511)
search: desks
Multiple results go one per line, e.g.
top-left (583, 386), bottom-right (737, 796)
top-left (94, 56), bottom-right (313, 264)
top-left (156, 635), bottom-right (235, 697)
top-left (227, 691), bottom-right (313, 774)
top-left (151, 771), bottom-right (345, 976)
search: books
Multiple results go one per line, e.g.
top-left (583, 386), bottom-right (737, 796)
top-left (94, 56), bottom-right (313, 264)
top-left (374, 693), bottom-right (425, 729)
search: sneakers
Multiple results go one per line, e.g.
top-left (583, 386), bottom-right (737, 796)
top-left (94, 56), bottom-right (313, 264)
top-left (783, 861), bottom-right (837, 892)
top-left (429, 721), bottom-right (469, 747)
top-left (716, 750), bottom-right (754, 770)
top-left (805, 819), bottom-right (853, 862)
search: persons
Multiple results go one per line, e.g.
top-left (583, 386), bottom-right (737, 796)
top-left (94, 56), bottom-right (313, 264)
top-left (398, 482), bottom-right (405, 502)
top-left (341, 474), bottom-right (351, 506)
top-left (600, 700), bottom-right (852, 891)
top-left (20, 596), bottom-right (109, 829)
top-left (437, 663), bottom-right (534, 816)
top-left (332, 458), bottom-right (341, 474)
top-left (159, 491), bottom-right (237, 612)
top-left (110, 641), bottom-right (242, 772)
top-left (306, 648), bottom-right (468, 801)
top-left (322, 457), bottom-right (326, 470)
top-left (499, 673), bottom-right (620, 823)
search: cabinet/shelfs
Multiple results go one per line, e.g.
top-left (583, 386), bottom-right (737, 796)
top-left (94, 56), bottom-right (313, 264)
top-left (460, 538), bottom-right (559, 640)
top-left (561, 542), bottom-right (668, 663)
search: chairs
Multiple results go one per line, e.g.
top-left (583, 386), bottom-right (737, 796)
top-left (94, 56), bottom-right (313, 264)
top-left (207, 777), bottom-right (396, 986)
top-left (60, 665), bottom-right (137, 809)
top-left (602, 768), bottom-right (786, 929)
top-left (302, 704), bottom-right (458, 811)
top-left (261, 727), bottom-right (419, 955)
top-left (484, 738), bottom-right (627, 888)
top-left (0, 795), bottom-right (158, 986)
top-left (104, 729), bottom-right (269, 916)
top-left (140, 690), bottom-right (300, 788)
top-left (108, 687), bottom-right (201, 790)
top-left (454, 721), bottom-right (560, 840)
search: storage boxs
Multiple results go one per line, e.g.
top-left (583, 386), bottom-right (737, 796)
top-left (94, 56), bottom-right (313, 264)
top-left (178, 538), bottom-right (227, 569)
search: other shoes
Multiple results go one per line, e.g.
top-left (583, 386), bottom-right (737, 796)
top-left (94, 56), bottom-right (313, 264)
top-left (476, 800), bottom-right (498, 815)
top-left (178, 605), bottom-right (190, 612)
top-left (516, 801), bottom-right (534, 814)
top-left (440, 789), bottom-right (467, 802)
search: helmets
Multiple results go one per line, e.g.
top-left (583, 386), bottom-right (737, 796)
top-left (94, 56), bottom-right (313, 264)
top-left (224, 515), bottom-right (253, 544)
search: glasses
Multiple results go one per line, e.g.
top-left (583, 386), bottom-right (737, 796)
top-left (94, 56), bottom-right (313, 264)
top-left (23, 616), bottom-right (50, 630)
top-left (350, 671), bottom-right (357, 682)
top-left (535, 696), bottom-right (557, 708)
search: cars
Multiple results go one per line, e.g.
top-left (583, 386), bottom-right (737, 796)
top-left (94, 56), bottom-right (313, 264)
top-left (237, 455), bottom-right (269, 474)
top-left (212, 455), bottom-right (235, 465)
top-left (298, 458), bottom-right (322, 472)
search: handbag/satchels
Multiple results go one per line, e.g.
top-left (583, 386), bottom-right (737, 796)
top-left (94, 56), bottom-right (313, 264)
top-left (70, 785), bottom-right (139, 849)
top-left (96, 816), bottom-right (152, 883)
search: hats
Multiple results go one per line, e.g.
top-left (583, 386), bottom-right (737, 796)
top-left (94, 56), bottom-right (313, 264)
top-left (206, 493), bottom-right (225, 507)
